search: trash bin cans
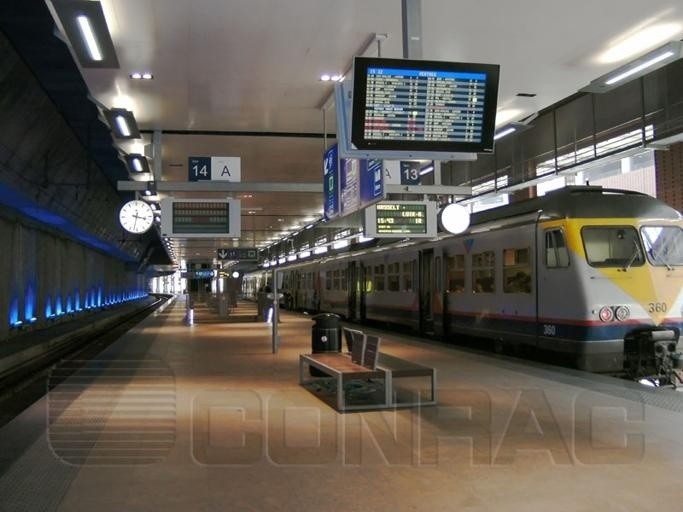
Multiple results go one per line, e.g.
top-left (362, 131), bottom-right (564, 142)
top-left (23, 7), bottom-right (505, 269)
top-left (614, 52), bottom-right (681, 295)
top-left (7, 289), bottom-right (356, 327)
top-left (309, 313), bottom-right (343, 377)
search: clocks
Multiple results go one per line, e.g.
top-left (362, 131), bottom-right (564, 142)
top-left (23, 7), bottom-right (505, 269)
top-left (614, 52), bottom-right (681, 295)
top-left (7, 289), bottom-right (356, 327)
top-left (118, 200), bottom-right (154, 234)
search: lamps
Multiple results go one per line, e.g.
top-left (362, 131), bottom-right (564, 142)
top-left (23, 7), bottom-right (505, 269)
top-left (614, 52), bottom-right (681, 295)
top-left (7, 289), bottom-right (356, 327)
top-left (578, 39), bottom-right (683, 95)
top-left (265, 216), bottom-right (328, 249)
top-left (147, 202), bottom-right (162, 224)
top-left (418, 162), bottom-right (434, 181)
top-left (51, 0), bottom-right (152, 174)
top-left (493, 111), bottom-right (538, 140)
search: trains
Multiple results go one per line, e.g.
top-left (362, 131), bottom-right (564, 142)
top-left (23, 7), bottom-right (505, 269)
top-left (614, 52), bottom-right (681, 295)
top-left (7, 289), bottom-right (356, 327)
top-left (242, 185), bottom-right (683, 386)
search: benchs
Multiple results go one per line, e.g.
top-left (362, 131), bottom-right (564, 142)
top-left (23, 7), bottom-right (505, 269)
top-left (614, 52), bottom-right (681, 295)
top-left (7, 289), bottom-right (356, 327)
top-left (377, 276), bottom-right (517, 293)
top-left (299, 325), bottom-right (438, 412)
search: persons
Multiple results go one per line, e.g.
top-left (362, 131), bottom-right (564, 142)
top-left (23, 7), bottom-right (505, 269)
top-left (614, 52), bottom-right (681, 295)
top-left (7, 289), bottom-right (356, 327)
top-left (504, 270), bottom-right (531, 294)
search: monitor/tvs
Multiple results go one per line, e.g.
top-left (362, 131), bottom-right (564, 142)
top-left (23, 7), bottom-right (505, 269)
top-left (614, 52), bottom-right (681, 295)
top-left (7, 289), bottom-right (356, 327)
top-left (350, 56), bottom-right (500, 156)
top-left (334, 79), bottom-right (480, 162)
